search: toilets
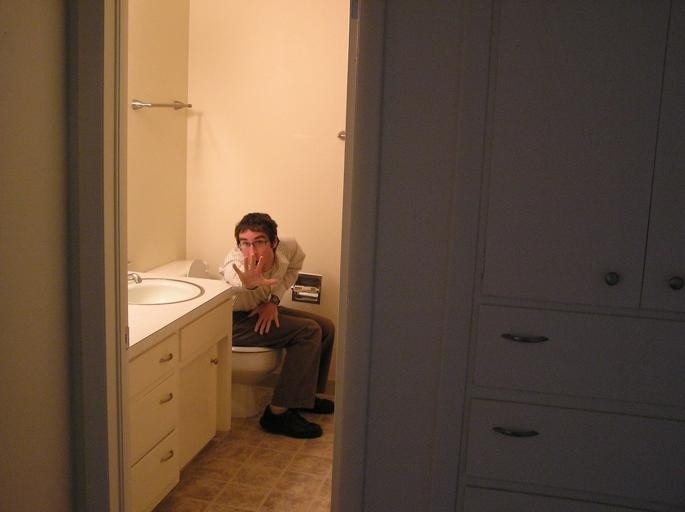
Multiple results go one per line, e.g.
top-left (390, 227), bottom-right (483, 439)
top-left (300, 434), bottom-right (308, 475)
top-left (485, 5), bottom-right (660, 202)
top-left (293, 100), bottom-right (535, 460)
top-left (147, 260), bottom-right (281, 419)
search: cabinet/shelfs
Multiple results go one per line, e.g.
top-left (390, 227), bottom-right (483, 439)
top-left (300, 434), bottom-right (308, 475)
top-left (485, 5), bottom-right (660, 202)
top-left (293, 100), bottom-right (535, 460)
top-left (433, 1), bottom-right (685, 512)
top-left (124, 300), bottom-right (231, 512)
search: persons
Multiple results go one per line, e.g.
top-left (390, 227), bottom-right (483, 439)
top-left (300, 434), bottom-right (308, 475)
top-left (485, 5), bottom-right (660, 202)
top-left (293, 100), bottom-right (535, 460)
top-left (218, 212), bottom-right (334, 439)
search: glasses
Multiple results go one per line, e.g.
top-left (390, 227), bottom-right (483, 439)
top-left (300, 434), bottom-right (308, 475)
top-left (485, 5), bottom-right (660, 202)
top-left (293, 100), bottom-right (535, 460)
top-left (238, 240), bottom-right (270, 249)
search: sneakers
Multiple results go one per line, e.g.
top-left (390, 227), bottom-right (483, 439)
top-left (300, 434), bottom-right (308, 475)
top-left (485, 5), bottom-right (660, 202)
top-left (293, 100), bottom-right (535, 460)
top-left (259, 404), bottom-right (323, 439)
top-left (288, 396), bottom-right (333, 414)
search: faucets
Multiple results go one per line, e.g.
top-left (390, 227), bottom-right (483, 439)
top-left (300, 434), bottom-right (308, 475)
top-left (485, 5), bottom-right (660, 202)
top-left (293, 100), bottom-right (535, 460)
top-left (128, 273), bottom-right (143, 284)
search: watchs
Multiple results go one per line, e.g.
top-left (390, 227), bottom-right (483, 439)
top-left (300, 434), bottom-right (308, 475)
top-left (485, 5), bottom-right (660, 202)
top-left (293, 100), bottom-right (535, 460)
top-left (270, 295), bottom-right (280, 305)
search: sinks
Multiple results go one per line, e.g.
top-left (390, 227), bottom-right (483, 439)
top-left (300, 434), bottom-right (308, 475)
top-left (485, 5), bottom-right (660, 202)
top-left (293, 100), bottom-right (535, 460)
top-left (128, 278), bottom-right (205, 306)
